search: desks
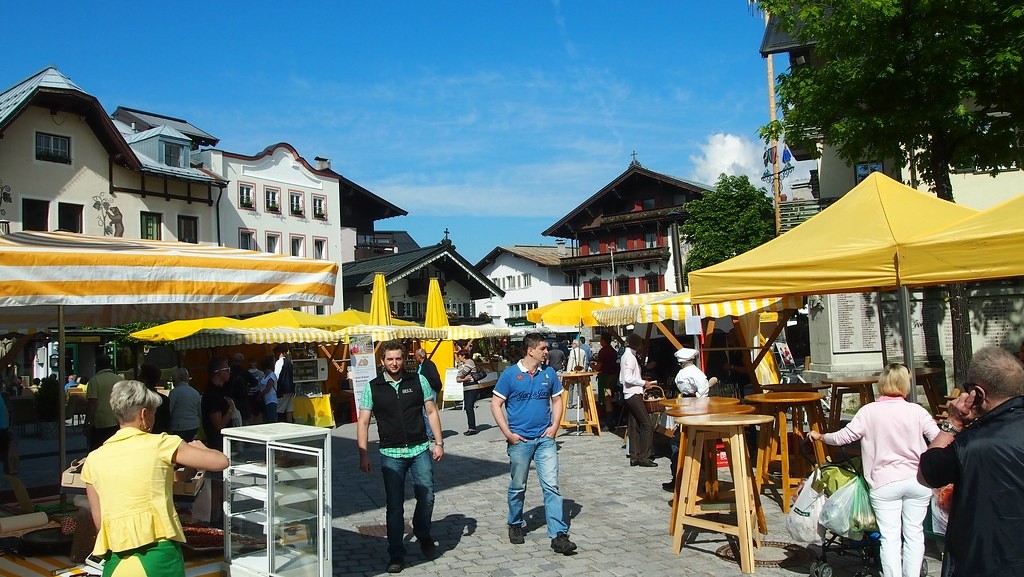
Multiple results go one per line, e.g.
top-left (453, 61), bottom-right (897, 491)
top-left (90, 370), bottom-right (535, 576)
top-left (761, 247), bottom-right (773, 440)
top-left (660, 377), bottom-right (880, 573)
top-left (557, 372), bottom-right (603, 438)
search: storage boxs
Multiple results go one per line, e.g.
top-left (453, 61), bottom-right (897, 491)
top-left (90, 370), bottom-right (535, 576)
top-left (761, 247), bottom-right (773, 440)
top-left (61, 457), bottom-right (206, 502)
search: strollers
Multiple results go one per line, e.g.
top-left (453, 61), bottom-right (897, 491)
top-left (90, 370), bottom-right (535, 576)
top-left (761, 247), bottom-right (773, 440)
top-left (798, 434), bottom-right (929, 577)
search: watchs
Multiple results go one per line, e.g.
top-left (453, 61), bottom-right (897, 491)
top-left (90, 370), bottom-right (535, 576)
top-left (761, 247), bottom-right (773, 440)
top-left (941, 420), bottom-right (959, 434)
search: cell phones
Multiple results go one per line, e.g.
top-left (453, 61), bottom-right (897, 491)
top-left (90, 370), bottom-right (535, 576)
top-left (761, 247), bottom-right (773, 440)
top-left (969, 387), bottom-right (983, 406)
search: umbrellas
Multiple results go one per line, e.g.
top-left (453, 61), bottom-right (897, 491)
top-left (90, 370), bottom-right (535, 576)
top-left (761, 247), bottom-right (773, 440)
top-left (527, 299), bottom-right (620, 436)
top-left (369, 271), bottom-right (393, 326)
top-left (322, 307), bottom-right (421, 325)
top-left (241, 308), bottom-right (353, 329)
top-left (130, 316), bottom-right (274, 341)
top-left (421, 277), bottom-right (454, 391)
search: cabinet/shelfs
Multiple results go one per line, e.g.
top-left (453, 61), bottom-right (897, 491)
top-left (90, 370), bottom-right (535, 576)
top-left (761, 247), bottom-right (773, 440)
top-left (220, 423), bottom-right (332, 577)
top-left (403, 340), bottom-right (419, 372)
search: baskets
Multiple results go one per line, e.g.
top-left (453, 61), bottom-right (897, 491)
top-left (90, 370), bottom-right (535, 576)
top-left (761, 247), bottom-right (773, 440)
top-left (643, 385), bottom-right (667, 414)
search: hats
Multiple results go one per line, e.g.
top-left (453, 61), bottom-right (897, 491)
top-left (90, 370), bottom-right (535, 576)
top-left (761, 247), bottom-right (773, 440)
top-left (674, 348), bottom-right (700, 363)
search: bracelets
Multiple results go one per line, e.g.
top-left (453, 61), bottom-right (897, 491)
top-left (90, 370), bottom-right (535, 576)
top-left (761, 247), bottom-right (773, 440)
top-left (435, 441), bottom-right (444, 447)
top-left (819, 434), bottom-right (823, 440)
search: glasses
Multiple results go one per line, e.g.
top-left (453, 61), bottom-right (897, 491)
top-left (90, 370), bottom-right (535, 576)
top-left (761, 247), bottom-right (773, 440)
top-left (215, 367), bottom-right (231, 372)
top-left (961, 381), bottom-right (988, 394)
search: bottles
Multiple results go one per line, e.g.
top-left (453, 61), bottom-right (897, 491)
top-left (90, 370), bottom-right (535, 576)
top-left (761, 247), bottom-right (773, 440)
top-left (708, 382), bottom-right (741, 399)
top-left (659, 377), bottom-right (690, 398)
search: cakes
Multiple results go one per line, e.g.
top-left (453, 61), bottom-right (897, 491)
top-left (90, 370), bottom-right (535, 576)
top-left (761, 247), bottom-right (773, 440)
top-left (275, 455), bottom-right (306, 468)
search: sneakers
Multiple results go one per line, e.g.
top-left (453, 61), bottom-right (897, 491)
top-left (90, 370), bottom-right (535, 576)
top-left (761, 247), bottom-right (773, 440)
top-left (550, 531), bottom-right (577, 552)
top-left (508, 524), bottom-right (525, 544)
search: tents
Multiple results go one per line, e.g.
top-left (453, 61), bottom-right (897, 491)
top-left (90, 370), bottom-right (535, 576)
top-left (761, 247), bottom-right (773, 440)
top-left (589, 290), bottom-right (676, 308)
top-left (0, 228), bottom-right (339, 509)
top-left (336, 324), bottom-right (447, 361)
top-left (448, 323), bottom-right (557, 372)
top-left (591, 290), bottom-right (803, 369)
top-left (687, 171), bottom-right (1024, 402)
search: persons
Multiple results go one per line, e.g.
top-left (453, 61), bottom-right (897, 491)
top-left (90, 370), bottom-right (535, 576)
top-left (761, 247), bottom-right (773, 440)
top-left (0, 345), bottom-right (298, 577)
top-left (80, 380), bottom-right (229, 577)
top-left (662, 348), bottom-right (709, 492)
top-left (415, 348), bottom-right (443, 443)
top-left (807, 363), bottom-right (940, 577)
top-left (917, 344), bottom-right (1024, 577)
top-left (358, 342), bottom-right (444, 573)
top-left (548, 332), bottom-right (659, 467)
top-left (490, 332), bottom-right (577, 553)
top-left (456, 349), bottom-right (479, 436)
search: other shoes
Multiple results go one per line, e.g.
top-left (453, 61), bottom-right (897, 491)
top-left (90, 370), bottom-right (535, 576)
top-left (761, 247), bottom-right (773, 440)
top-left (463, 431), bottom-right (478, 436)
top-left (387, 558), bottom-right (404, 573)
top-left (639, 461), bottom-right (659, 467)
top-left (574, 406), bottom-right (581, 409)
top-left (566, 406), bottom-right (572, 408)
top-left (662, 480), bottom-right (674, 493)
top-left (413, 528), bottom-right (435, 557)
top-left (630, 461), bottom-right (639, 466)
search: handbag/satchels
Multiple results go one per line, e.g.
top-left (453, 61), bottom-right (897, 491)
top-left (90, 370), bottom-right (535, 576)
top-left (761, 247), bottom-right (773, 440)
top-left (820, 475), bottom-right (864, 542)
top-left (467, 366), bottom-right (487, 384)
top-left (3, 432), bottom-right (19, 474)
top-left (785, 467), bottom-right (826, 547)
top-left (849, 472), bottom-right (880, 533)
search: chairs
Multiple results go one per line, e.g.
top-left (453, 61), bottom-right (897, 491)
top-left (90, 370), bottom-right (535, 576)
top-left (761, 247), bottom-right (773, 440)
top-left (9, 398), bottom-right (85, 439)
